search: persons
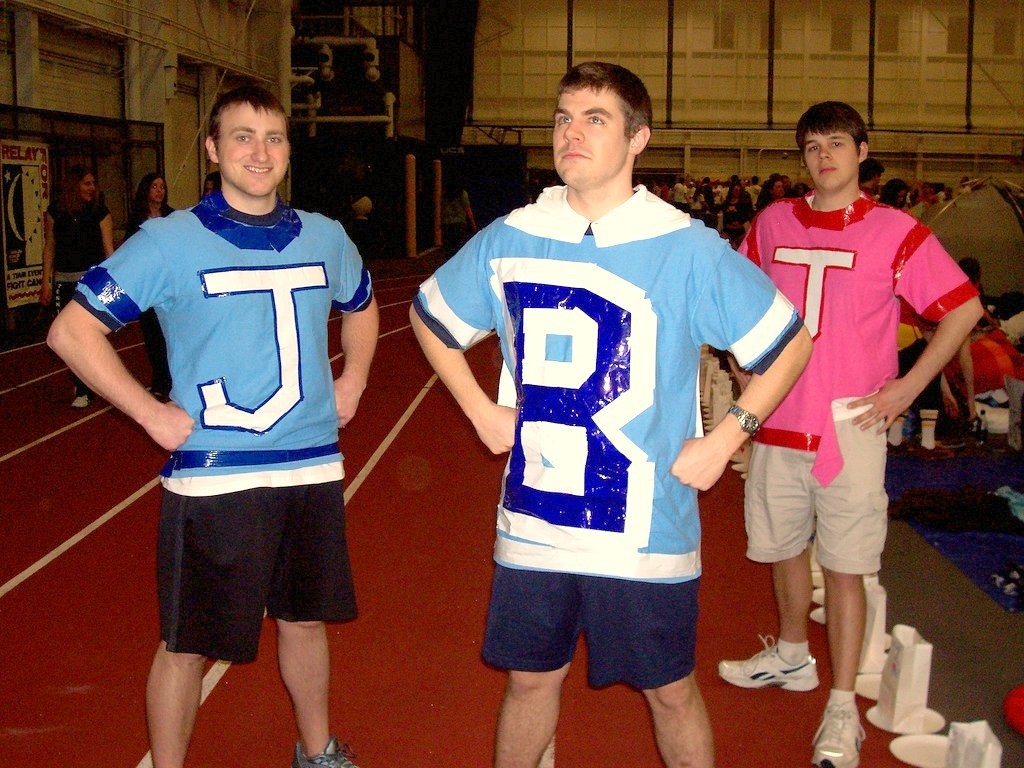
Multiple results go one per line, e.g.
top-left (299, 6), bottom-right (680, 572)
top-left (40, 163), bottom-right (114, 408)
top-left (859, 158), bottom-right (1024, 433)
top-left (45, 86), bottom-right (378, 768)
top-left (122, 171), bottom-right (175, 399)
top-left (440, 176), bottom-right (477, 258)
top-left (408, 60), bottom-right (814, 768)
top-left (633, 172), bottom-right (811, 250)
top-left (202, 170), bottom-right (222, 196)
top-left (726, 102), bottom-right (986, 768)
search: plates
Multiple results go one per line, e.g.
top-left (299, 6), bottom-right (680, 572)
top-left (729, 453), bottom-right (749, 479)
top-left (854, 674), bottom-right (914, 700)
top-left (808, 544), bottom-right (879, 624)
top-left (866, 705), bottom-right (946, 735)
top-left (889, 735), bottom-right (948, 768)
top-left (699, 391), bottom-right (714, 439)
top-left (883, 633), bottom-right (891, 662)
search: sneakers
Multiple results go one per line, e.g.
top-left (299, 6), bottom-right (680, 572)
top-left (719, 636), bottom-right (819, 692)
top-left (72, 394), bottom-right (90, 408)
top-left (292, 736), bottom-right (360, 768)
top-left (810, 700), bottom-right (865, 768)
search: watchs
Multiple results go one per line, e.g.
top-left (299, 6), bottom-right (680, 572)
top-left (728, 405), bottom-right (760, 436)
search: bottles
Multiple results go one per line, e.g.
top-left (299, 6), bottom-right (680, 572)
top-left (977, 409), bottom-right (988, 445)
